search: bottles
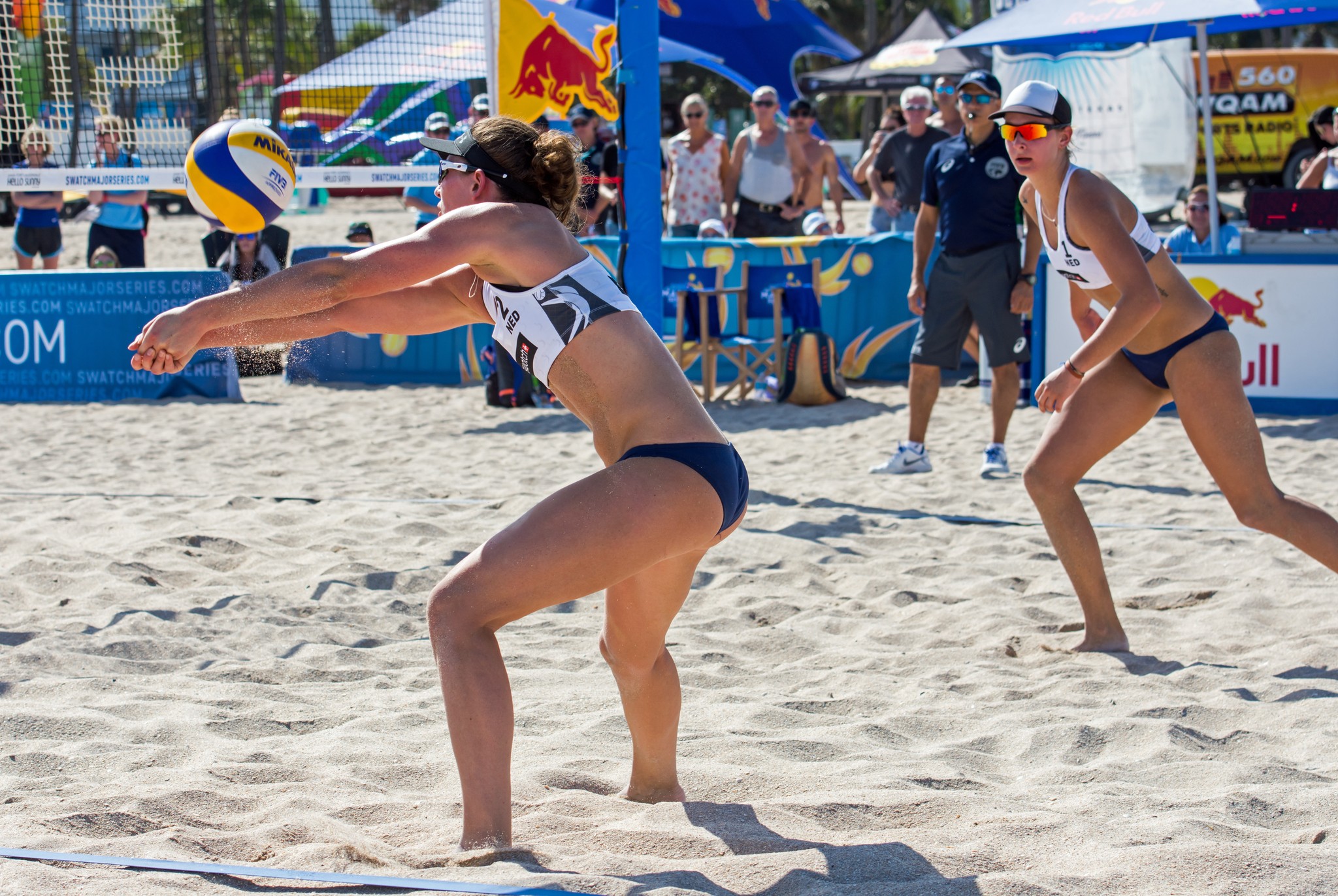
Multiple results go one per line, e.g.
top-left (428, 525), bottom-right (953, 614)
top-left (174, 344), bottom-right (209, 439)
top-left (1225, 236), bottom-right (1241, 256)
top-left (754, 370), bottom-right (767, 401)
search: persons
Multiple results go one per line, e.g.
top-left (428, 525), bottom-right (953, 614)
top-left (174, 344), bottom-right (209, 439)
top-left (659, 74), bottom-right (1029, 387)
top-left (155, 94), bottom-right (625, 376)
top-left (85, 115), bottom-right (152, 267)
top-left (989, 81), bottom-right (1338, 655)
top-left (10, 123), bottom-right (64, 270)
top-left (128, 116), bottom-right (750, 867)
top-left (1277, 105), bottom-right (1338, 191)
top-left (1158, 184), bottom-right (1243, 257)
top-left (869, 68), bottom-right (1042, 479)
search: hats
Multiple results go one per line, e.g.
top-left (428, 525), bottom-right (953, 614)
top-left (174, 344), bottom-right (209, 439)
top-left (568, 104), bottom-right (596, 126)
top-left (788, 100), bottom-right (811, 117)
top-left (531, 116), bottom-right (549, 127)
top-left (801, 212), bottom-right (833, 235)
top-left (419, 128), bottom-right (542, 193)
top-left (988, 81), bottom-right (1072, 124)
top-left (424, 112), bottom-right (450, 130)
top-left (472, 93), bottom-right (489, 110)
top-left (698, 218), bottom-right (730, 239)
top-left (347, 222), bottom-right (373, 241)
top-left (956, 71), bottom-right (1001, 98)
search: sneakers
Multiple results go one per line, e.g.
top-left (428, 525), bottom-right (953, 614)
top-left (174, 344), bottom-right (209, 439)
top-left (981, 446), bottom-right (1010, 478)
top-left (867, 439), bottom-right (933, 474)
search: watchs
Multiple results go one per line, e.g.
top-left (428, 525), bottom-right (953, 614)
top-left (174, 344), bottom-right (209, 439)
top-left (1017, 271), bottom-right (1037, 286)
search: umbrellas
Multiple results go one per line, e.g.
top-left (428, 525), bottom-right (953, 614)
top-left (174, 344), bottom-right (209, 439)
top-left (934, 0), bottom-right (1337, 253)
top-left (273, 0), bottom-right (724, 117)
top-left (558, 1), bottom-right (865, 201)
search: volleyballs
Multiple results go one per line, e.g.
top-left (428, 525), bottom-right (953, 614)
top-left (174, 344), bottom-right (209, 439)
top-left (184, 118), bottom-right (296, 234)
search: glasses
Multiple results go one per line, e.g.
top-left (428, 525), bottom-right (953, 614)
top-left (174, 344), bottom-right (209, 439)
top-left (686, 112), bottom-right (702, 118)
top-left (813, 224), bottom-right (830, 235)
top-left (875, 87), bottom-right (1071, 142)
top-left (237, 234), bottom-right (254, 241)
top-left (573, 121), bottom-right (587, 127)
top-left (755, 100), bottom-right (773, 107)
top-left (95, 260), bottom-right (114, 268)
top-left (792, 112), bottom-right (809, 118)
top-left (1185, 203), bottom-right (1210, 212)
top-left (438, 159), bottom-right (508, 185)
top-left (702, 232), bottom-right (720, 238)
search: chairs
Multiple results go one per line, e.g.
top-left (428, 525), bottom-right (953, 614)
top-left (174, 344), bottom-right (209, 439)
top-left (661, 265), bottom-right (722, 401)
top-left (200, 225), bottom-right (289, 279)
top-left (697, 258), bottom-right (820, 406)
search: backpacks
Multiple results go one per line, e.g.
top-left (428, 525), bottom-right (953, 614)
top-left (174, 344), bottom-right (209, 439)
top-left (776, 327), bottom-right (846, 407)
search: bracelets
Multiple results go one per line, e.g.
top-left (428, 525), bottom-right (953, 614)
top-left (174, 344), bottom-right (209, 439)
top-left (1063, 358), bottom-right (1086, 383)
top-left (103, 194), bottom-right (108, 203)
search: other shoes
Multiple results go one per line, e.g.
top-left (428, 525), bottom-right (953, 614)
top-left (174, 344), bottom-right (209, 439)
top-left (958, 373), bottom-right (980, 389)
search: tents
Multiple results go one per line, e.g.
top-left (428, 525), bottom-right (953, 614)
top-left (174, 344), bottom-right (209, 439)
top-left (788, 7), bottom-right (992, 133)
